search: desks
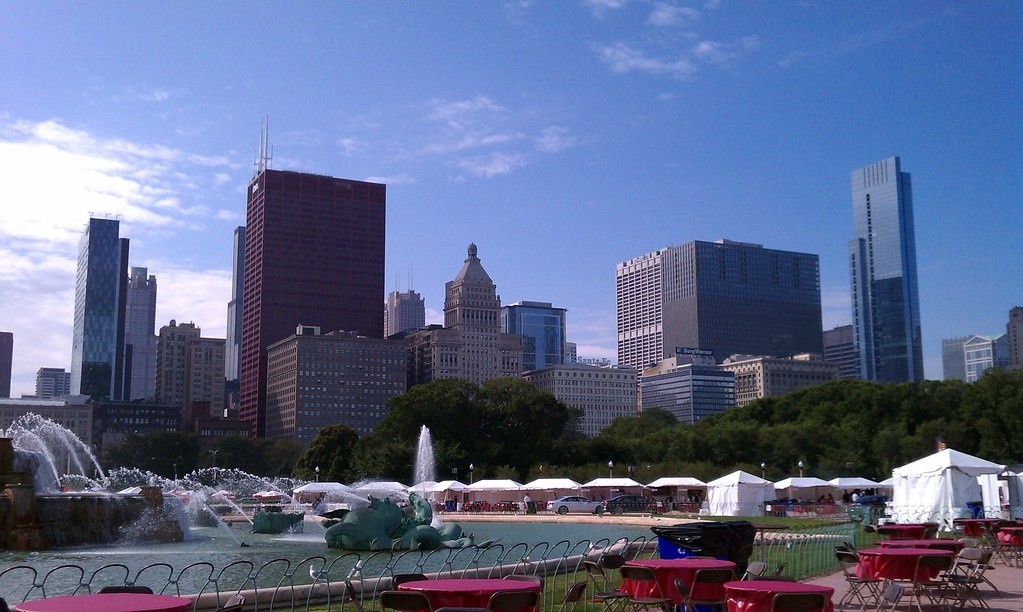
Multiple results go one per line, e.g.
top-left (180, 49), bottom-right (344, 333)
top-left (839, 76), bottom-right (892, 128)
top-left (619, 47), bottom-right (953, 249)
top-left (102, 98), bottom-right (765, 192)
top-left (854, 547), bottom-right (954, 612)
top-left (963, 519), bottom-right (1007, 539)
top-left (619, 558), bottom-right (738, 612)
top-left (880, 521), bottom-right (940, 540)
top-left (398, 579), bottom-right (540, 612)
top-left (723, 581), bottom-right (835, 612)
top-left (15, 594), bottom-right (193, 612)
top-left (996, 527), bottom-right (1023, 568)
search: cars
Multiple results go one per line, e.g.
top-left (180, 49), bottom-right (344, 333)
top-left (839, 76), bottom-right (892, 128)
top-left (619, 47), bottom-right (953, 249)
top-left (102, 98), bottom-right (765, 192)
top-left (848, 495), bottom-right (887, 521)
top-left (118, 487), bottom-right (142, 496)
top-left (253, 490), bottom-right (286, 499)
top-left (211, 490), bottom-right (237, 501)
top-left (602, 495), bottom-right (657, 513)
top-left (167, 487), bottom-right (195, 500)
top-left (546, 494), bottom-right (604, 516)
top-left (884, 498), bottom-right (893, 517)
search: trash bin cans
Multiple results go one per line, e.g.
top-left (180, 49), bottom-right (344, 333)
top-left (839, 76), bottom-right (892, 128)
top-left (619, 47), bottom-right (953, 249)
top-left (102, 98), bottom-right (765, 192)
top-left (445, 501), bottom-right (456, 512)
top-left (763, 499), bottom-right (786, 518)
top-left (527, 500), bottom-right (536, 515)
top-left (856, 495), bottom-right (889, 527)
top-left (649, 520), bottom-right (756, 611)
top-left (966, 501), bottom-right (984, 518)
top-left (788, 499), bottom-right (798, 511)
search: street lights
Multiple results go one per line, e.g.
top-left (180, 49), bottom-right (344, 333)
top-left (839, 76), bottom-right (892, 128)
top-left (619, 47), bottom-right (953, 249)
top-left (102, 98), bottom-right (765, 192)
top-left (208, 448), bottom-right (219, 466)
top-left (761, 462), bottom-right (766, 479)
top-left (469, 463), bottom-right (474, 484)
top-left (315, 466), bottom-right (320, 483)
top-left (608, 461), bottom-right (614, 478)
top-left (798, 459), bottom-right (805, 477)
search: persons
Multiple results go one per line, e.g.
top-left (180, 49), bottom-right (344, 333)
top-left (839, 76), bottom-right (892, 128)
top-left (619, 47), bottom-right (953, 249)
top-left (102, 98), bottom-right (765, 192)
top-left (842, 489), bottom-right (851, 503)
top-left (444, 495), bottom-right (449, 511)
top-left (299, 495), bottom-right (304, 504)
top-left (524, 493), bottom-right (530, 506)
top-left (695, 492), bottom-right (700, 513)
top-left (667, 495), bottom-right (675, 510)
top-left (827, 493), bottom-right (835, 504)
top-left (818, 495), bottom-right (825, 503)
top-left (313, 496), bottom-right (321, 511)
top-left (777, 497), bottom-right (802, 511)
top-left (851, 490), bottom-right (859, 502)
top-left (452, 495), bottom-right (458, 510)
top-left (553, 493), bottom-right (557, 501)
top-left (319, 498), bottom-right (326, 514)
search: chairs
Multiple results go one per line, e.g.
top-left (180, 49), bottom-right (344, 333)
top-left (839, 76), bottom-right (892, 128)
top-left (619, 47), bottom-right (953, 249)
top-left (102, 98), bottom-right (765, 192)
top-left (674, 577), bottom-right (697, 612)
top-left (392, 574), bottom-right (429, 591)
top-left (559, 581), bottom-right (587, 612)
top-left (772, 564), bottom-right (784, 577)
top-left (832, 518), bottom-right (1023, 612)
top-left (487, 589), bottom-right (539, 612)
top-left (582, 560), bottom-right (629, 612)
top-left (96, 585), bottom-right (153, 594)
top-left (740, 562), bottom-right (767, 581)
top-left (344, 580), bottom-right (380, 612)
top-left (619, 565), bottom-right (674, 612)
top-left (222, 593), bottom-right (246, 608)
top-left (752, 575), bottom-right (797, 583)
top-left (378, 591), bottom-right (432, 612)
top-left (598, 554), bottom-right (640, 612)
top-left (770, 593), bottom-right (826, 612)
top-left (501, 574), bottom-right (552, 607)
top-left (684, 569), bottom-right (734, 612)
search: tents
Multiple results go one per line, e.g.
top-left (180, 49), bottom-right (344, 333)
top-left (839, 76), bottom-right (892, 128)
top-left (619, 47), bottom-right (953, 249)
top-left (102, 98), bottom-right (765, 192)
top-left (774, 478), bottom-right (834, 503)
top-left (581, 478), bottom-right (645, 501)
top-left (463, 480), bottom-right (523, 504)
top-left (830, 478), bottom-right (892, 501)
top-left (706, 470), bottom-right (777, 516)
top-left (646, 478), bottom-right (707, 502)
top-left (892, 449), bottom-right (1008, 525)
top-left (520, 479), bottom-right (582, 502)
top-left (293, 480), bottom-right (467, 508)
